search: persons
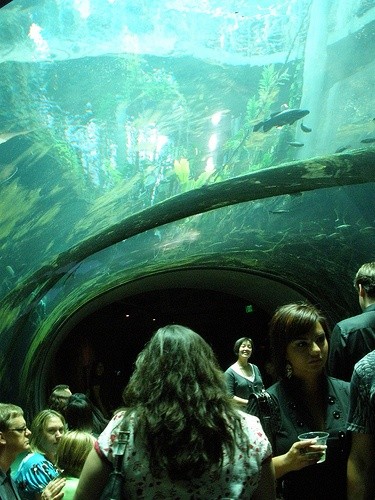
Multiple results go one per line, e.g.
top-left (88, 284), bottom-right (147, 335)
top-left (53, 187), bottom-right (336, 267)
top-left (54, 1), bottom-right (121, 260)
top-left (226, 336), bottom-right (268, 415)
top-left (70, 392), bottom-right (114, 427)
top-left (48, 391), bottom-right (72, 416)
top-left (245, 301), bottom-right (352, 500)
top-left (54, 385), bottom-right (73, 399)
top-left (52, 431), bottom-right (98, 500)
top-left (63, 400), bottom-right (102, 440)
top-left (344, 348), bottom-right (375, 499)
top-left (262, 361), bottom-right (283, 389)
top-left (0, 403), bottom-right (66, 500)
top-left (72, 326), bottom-right (277, 500)
top-left (13, 409), bottom-right (68, 500)
top-left (54, 328), bottom-right (157, 412)
top-left (327, 262), bottom-right (375, 384)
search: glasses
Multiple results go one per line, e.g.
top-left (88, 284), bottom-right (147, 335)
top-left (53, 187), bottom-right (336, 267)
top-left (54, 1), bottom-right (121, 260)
top-left (5, 425), bottom-right (30, 433)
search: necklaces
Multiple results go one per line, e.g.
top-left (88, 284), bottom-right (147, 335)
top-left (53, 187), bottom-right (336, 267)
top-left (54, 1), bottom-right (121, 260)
top-left (239, 365), bottom-right (249, 370)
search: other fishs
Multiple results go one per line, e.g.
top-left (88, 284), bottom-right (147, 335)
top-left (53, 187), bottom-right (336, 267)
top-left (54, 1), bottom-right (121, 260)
top-left (335, 145), bottom-right (351, 153)
top-left (285, 142), bottom-right (302, 147)
top-left (300, 123), bottom-right (310, 132)
top-left (272, 208), bottom-right (290, 215)
top-left (253, 108), bottom-right (310, 132)
top-left (362, 137), bottom-right (374, 143)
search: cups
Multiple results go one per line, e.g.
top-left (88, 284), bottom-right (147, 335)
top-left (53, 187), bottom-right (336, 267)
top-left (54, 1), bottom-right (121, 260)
top-left (297, 431), bottom-right (330, 465)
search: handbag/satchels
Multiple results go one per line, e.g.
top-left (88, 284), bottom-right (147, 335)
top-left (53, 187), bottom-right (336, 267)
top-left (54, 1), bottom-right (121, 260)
top-left (95, 408), bottom-right (132, 500)
